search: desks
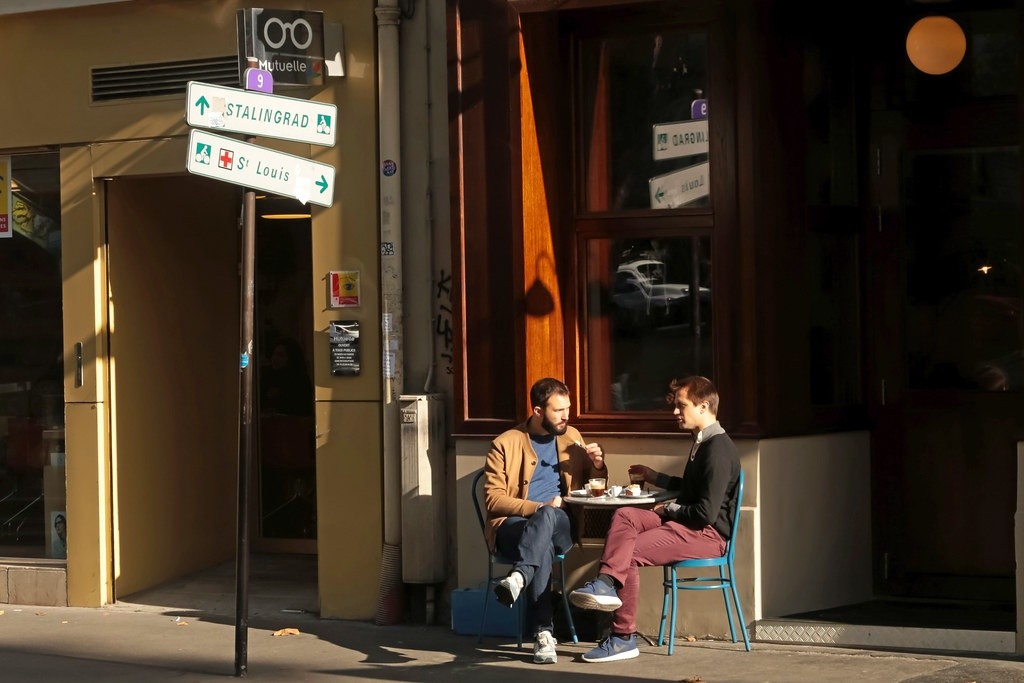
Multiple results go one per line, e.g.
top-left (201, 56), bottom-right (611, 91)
top-left (563, 492), bottom-right (681, 647)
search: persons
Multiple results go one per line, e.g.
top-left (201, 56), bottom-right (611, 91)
top-left (569, 376), bottom-right (741, 663)
top-left (266, 338), bottom-right (313, 415)
top-left (646, 239), bottom-right (673, 284)
top-left (483, 378), bottom-right (608, 663)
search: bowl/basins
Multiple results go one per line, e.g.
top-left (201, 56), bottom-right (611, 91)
top-left (625, 489), bottom-right (641, 497)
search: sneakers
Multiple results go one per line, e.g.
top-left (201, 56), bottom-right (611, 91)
top-left (494, 576), bottom-right (519, 609)
top-left (569, 580), bottom-right (622, 612)
top-left (581, 634), bottom-right (640, 662)
top-left (533, 630), bottom-right (557, 664)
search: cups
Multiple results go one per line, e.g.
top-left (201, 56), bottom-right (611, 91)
top-left (630, 472), bottom-right (645, 490)
top-left (584, 484), bottom-right (592, 497)
top-left (589, 478), bottom-right (606, 497)
top-left (607, 485), bottom-right (622, 497)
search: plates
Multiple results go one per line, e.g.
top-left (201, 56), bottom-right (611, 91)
top-left (569, 489), bottom-right (608, 496)
top-left (620, 489), bottom-right (662, 498)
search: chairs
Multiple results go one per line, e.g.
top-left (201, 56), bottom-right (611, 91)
top-left (261, 415), bottom-right (317, 539)
top-left (656, 471), bottom-right (751, 655)
top-left (472, 471), bottom-right (579, 652)
top-left (0, 415), bottom-right (51, 546)
